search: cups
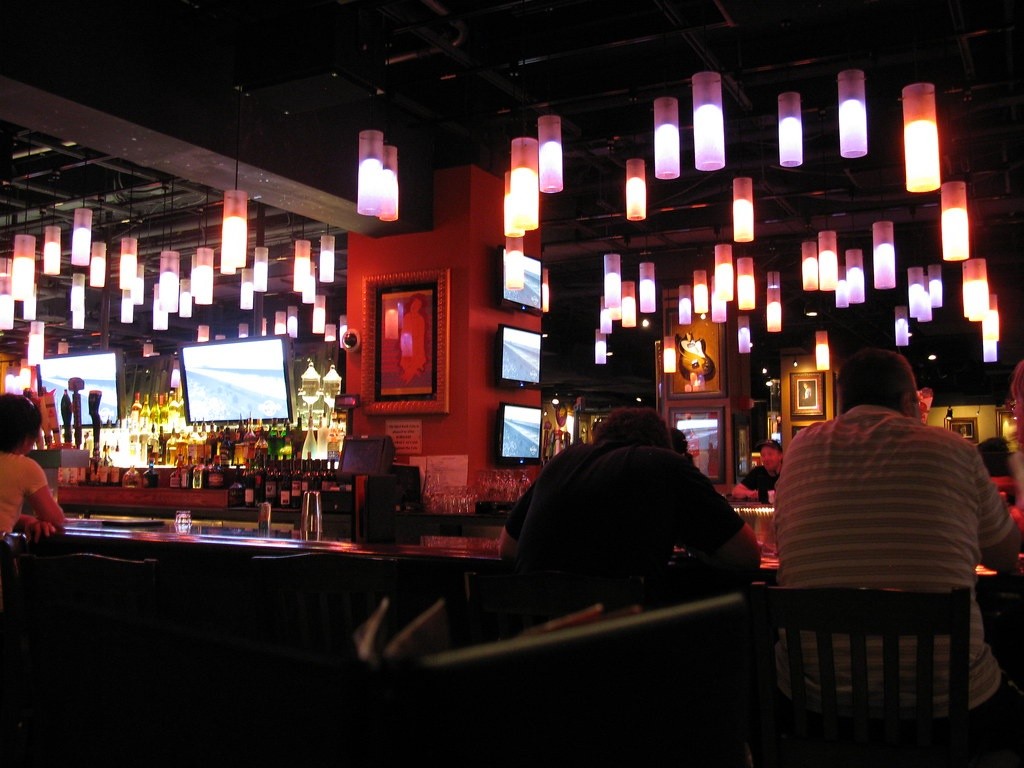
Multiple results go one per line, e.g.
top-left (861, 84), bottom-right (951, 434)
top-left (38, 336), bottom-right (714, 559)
top-left (174, 511), bottom-right (191, 535)
top-left (300, 491), bottom-right (323, 540)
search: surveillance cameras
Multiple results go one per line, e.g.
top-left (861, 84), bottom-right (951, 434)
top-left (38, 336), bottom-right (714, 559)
top-left (342, 328), bottom-right (361, 354)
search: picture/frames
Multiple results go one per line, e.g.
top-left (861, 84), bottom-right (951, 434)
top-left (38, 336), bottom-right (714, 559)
top-left (943, 416), bottom-right (978, 444)
top-left (667, 305), bottom-right (730, 398)
top-left (789, 372), bottom-right (827, 417)
top-left (996, 410), bottom-right (1017, 438)
top-left (792, 424), bottom-right (806, 438)
top-left (669, 404), bottom-right (727, 486)
top-left (359, 268), bottom-right (454, 414)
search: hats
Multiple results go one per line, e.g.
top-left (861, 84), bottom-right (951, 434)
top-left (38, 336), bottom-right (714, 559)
top-left (757, 439), bottom-right (782, 451)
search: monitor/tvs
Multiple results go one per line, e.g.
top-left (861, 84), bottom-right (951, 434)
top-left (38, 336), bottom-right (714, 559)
top-left (177, 333), bottom-right (295, 427)
top-left (337, 435), bottom-right (396, 477)
top-left (495, 324), bottom-right (543, 390)
top-left (495, 402), bottom-right (543, 466)
top-left (496, 245), bottom-right (543, 317)
top-left (35, 349), bottom-right (126, 429)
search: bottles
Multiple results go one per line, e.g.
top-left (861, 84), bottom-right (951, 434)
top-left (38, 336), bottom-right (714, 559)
top-left (98, 361), bottom-right (352, 511)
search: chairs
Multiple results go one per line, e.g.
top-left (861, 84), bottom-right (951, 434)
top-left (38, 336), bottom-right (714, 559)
top-left (1, 538), bottom-right (1024, 768)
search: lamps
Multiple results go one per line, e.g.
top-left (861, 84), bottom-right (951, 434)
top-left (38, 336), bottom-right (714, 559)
top-left (0, 126), bottom-right (402, 398)
top-left (504, 64), bottom-right (1008, 370)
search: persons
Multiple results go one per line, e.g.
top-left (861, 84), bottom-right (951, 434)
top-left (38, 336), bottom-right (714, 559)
top-left (767, 344), bottom-right (1024, 752)
top-left (732, 440), bottom-right (785, 503)
top-left (0, 392), bottom-right (66, 617)
top-left (495, 407), bottom-right (762, 580)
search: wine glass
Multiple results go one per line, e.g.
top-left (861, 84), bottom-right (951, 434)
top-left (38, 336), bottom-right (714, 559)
top-left (422, 469), bottom-right (531, 512)
